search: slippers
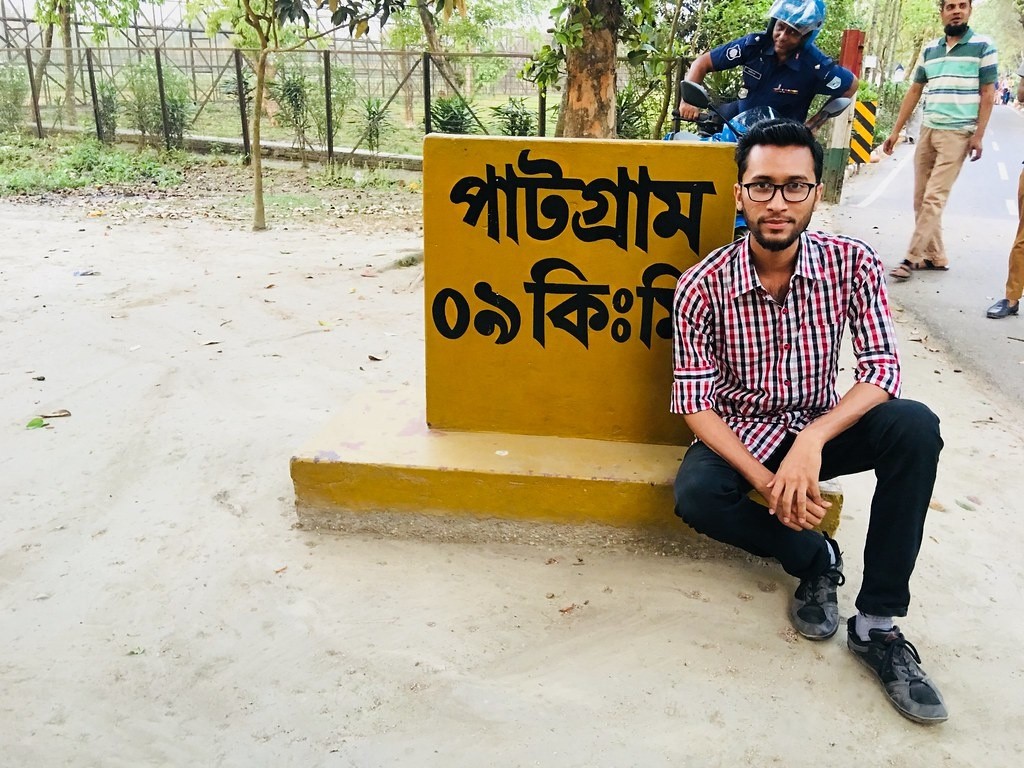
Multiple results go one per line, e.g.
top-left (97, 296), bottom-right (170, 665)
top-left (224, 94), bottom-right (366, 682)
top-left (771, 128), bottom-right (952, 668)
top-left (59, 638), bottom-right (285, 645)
top-left (889, 267), bottom-right (913, 279)
top-left (912, 259), bottom-right (950, 270)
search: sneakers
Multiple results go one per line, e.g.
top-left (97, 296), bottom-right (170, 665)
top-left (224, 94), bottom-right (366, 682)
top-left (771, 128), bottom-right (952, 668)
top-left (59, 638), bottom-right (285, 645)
top-left (791, 537), bottom-right (843, 642)
top-left (847, 618), bottom-right (949, 724)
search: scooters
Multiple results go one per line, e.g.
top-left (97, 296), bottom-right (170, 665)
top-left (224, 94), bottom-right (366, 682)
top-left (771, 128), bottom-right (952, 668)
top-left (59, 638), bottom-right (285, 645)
top-left (663, 78), bottom-right (851, 245)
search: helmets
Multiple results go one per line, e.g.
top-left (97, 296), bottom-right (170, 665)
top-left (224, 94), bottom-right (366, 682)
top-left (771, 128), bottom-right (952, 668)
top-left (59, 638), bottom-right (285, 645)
top-left (767, 0), bottom-right (826, 53)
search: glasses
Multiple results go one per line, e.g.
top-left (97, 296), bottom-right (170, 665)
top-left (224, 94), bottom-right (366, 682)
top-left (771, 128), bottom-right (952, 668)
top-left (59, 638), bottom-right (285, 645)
top-left (741, 181), bottom-right (818, 202)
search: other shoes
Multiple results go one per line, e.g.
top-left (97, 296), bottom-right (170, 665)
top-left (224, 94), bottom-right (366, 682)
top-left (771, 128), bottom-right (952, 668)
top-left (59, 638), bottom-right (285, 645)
top-left (987, 299), bottom-right (1019, 318)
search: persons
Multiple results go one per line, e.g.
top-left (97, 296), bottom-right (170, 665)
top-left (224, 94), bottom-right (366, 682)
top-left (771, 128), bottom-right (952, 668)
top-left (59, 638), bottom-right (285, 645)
top-left (666, 122), bottom-right (952, 728)
top-left (993, 75), bottom-right (1013, 104)
top-left (883, 0), bottom-right (999, 281)
top-left (679, 1), bottom-right (859, 145)
top-left (985, 59), bottom-right (1024, 320)
